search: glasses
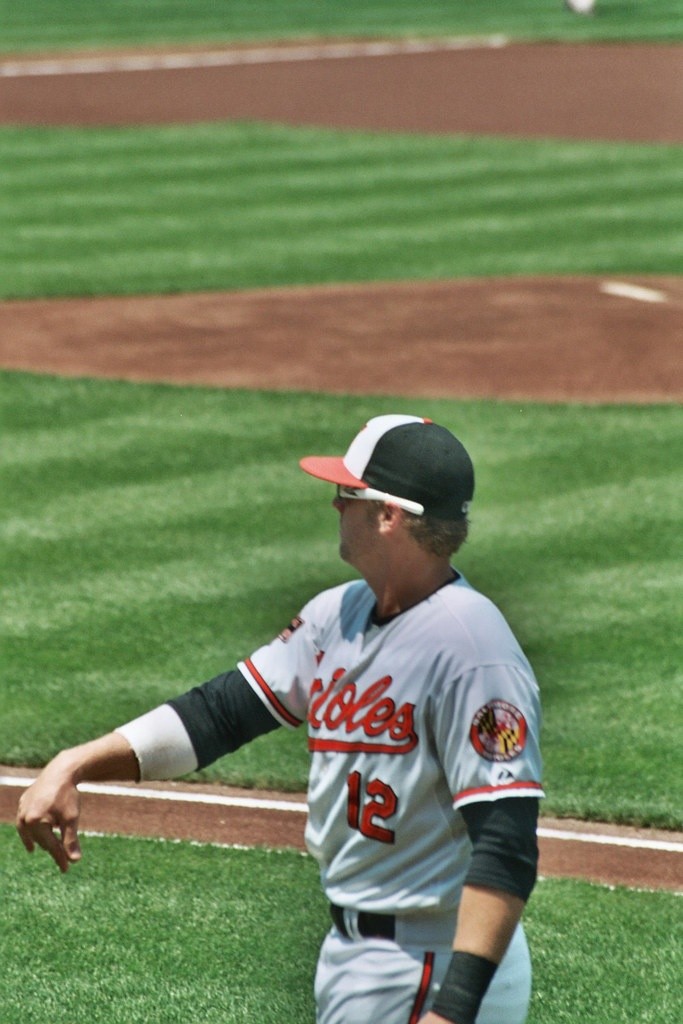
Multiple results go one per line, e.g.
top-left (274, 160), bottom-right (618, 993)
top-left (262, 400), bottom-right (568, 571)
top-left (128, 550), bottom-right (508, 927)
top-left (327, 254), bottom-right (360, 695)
top-left (336, 483), bottom-right (424, 516)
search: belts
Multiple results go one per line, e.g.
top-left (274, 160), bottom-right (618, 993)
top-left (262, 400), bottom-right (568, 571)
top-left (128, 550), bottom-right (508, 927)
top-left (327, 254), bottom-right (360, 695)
top-left (329, 902), bottom-right (395, 942)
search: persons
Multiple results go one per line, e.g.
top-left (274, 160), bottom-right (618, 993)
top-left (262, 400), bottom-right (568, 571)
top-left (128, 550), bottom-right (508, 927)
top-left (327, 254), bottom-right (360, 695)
top-left (15, 414), bottom-right (548, 1024)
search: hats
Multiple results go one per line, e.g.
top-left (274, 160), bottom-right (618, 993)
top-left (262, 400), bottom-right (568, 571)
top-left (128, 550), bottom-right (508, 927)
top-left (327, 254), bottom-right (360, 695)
top-left (298, 414), bottom-right (475, 517)
